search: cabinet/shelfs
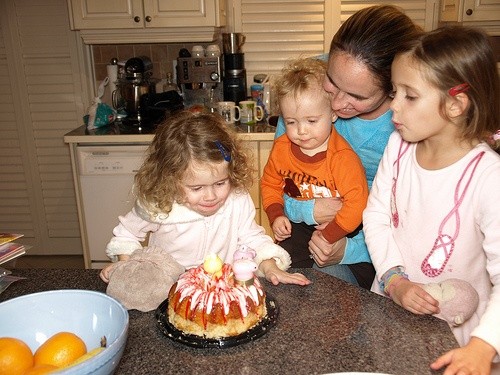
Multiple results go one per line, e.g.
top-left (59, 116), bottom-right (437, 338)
top-left (66, 0), bottom-right (228, 44)
top-left (69, 140), bottom-right (275, 269)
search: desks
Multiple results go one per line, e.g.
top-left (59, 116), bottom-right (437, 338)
top-left (0, 269), bottom-right (459, 375)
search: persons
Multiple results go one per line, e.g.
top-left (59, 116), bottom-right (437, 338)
top-left (261, 58), bottom-right (376, 291)
top-left (362, 26), bottom-right (500, 375)
top-left (274, 5), bottom-right (425, 287)
top-left (99, 110), bottom-right (311, 286)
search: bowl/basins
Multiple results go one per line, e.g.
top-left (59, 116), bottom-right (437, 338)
top-left (0, 289), bottom-right (130, 375)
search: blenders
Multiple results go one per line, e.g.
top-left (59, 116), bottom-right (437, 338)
top-left (222, 54), bottom-right (247, 106)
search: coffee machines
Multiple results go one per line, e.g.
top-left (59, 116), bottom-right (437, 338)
top-left (111, 57), bottom-right (157, 128)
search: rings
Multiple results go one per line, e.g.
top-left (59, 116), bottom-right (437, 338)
top-left (310, 252), bottom-right (315, 259)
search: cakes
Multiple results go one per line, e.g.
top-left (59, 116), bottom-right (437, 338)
top-left (168, 246), bottom-right (267, 339)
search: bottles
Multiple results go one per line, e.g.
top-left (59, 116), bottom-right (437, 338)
top-left (250, 74), bottom-right (270, 101)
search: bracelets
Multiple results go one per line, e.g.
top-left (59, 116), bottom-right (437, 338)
top-left (381, 265), bottom-right (409, 295)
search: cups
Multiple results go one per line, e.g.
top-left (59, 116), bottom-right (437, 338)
top-left (217, 100), bottom-right (242, 124)
top-left (222, 32), bottom-right (246, 54)
top-left (178, 44), bottom-right (222, 58)
top-left (238, 100), bottom-right (264, 124)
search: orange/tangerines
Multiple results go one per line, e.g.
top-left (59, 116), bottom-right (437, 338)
top-left (0, 331), bottom-right (110, 375)
